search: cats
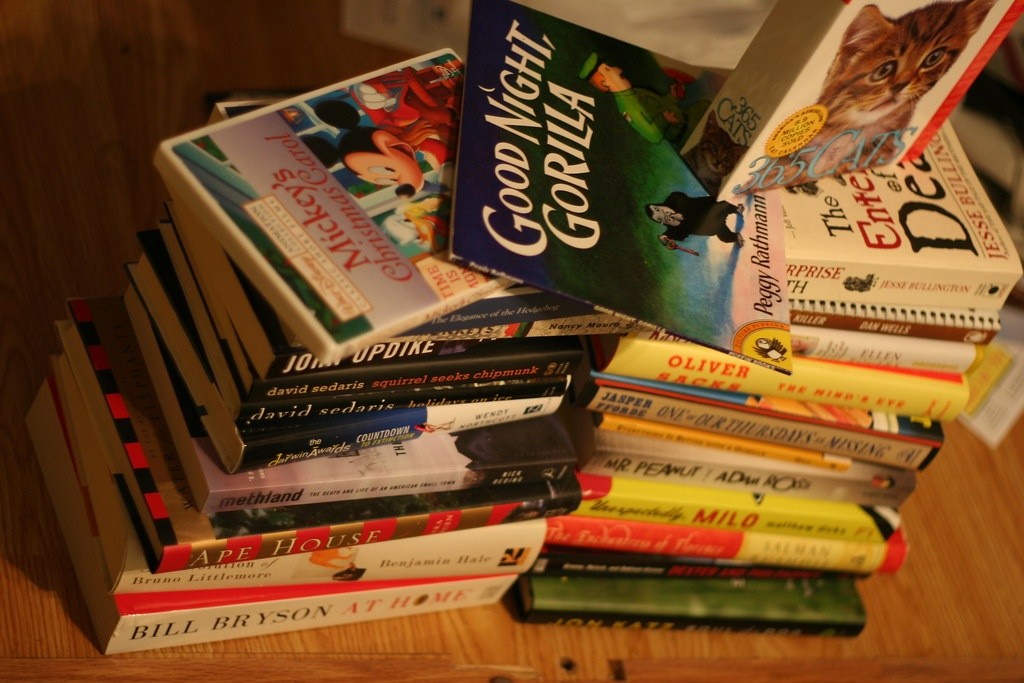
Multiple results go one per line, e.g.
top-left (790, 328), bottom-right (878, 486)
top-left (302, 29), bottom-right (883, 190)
top-left (681, 109), bottom-right (750, 199)
top-left (736, 0), bottom-right (997, 197)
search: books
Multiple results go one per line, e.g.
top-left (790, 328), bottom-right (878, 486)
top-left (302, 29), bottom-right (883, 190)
top-left (23, 0), bottom-right (1023, 655)
